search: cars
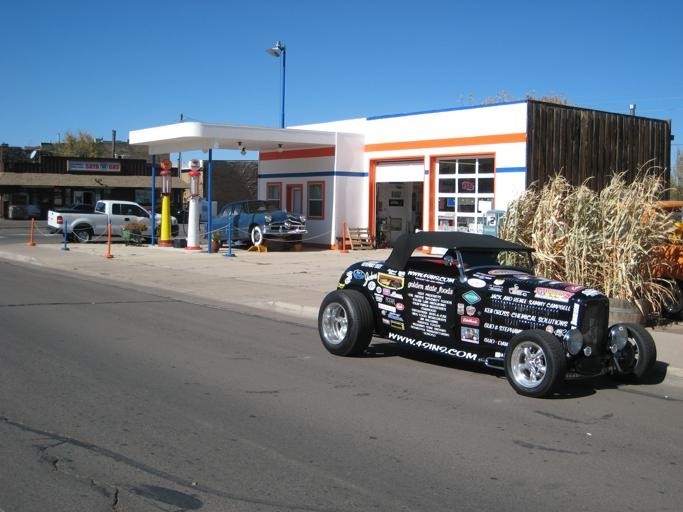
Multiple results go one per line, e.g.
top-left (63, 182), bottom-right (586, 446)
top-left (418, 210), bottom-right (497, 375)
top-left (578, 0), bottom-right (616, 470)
top-left (147, 204), bottom-right (201, 224)
top-left (203, 197), bottom-right (310, 248)
top-left (52, 202), bottom-right (95, 213)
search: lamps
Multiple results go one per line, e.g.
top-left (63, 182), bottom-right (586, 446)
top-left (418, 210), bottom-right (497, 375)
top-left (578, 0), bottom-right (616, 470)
top-left (240, 148), bottom-right (247, 155)
top-left (237, 142), bottom-right (243, 150)
top-left (277, 144), bottom-right (284, 153)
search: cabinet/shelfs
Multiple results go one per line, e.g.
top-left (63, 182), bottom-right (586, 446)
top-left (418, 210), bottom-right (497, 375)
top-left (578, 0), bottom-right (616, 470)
top-left (378, 224), bottom-right (392, 248)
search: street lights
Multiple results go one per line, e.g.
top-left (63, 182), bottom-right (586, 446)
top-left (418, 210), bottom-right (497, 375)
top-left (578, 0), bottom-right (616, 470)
top-left (264, 40), bottom-right (289, 129)
top-left (628, 102), bottom-right (637, 115)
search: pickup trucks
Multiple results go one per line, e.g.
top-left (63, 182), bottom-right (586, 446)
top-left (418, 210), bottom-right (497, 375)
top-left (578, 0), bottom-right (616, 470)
top-left (45, 199), bottom-right (181, 244)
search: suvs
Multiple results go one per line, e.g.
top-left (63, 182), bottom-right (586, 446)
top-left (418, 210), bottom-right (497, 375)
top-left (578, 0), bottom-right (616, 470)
top-left (310, 229), bottom-right (661, 398)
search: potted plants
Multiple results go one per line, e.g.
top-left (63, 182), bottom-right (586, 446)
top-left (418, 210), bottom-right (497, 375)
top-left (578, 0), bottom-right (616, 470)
top-left (120, 223), bottom-right (148, 246)
top-left (209, 229), bottom-right (225, 253)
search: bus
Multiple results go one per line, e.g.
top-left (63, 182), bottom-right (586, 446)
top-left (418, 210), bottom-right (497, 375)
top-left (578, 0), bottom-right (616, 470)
top-left (6, 191), bottom-right (29, 220)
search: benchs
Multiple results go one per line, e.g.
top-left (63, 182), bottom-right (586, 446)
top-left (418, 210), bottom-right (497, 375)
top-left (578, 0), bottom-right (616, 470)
top-left (343, 222), bottom-right (372, 251)
